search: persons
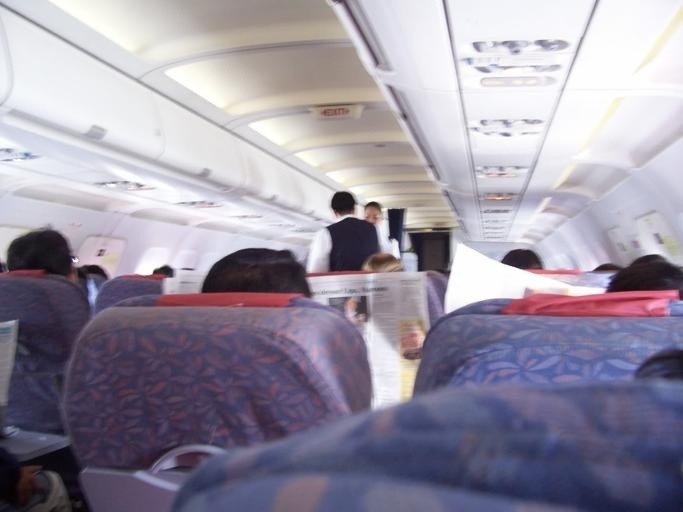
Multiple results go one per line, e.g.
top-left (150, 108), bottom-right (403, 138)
top-left (501, 249), bottom-right (542, 272)
top-left (0, 446), bottom-right (68, 511)
top-left (7, 229), bottom-right (74, 280)
top-left (604, 255), bottom-right (683, 298)
top-left (305, 191), bottom-right (379, 274)
top-left (363, 200), bottom-right (400, 259)
top-left (202, 246), bottom-right (313, 301)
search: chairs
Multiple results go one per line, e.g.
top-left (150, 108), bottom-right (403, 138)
top-left (62, 293), bottom-right (373, 512)
top-left (412, 290), bottom-right (682, 400)
top-left (306, 270), bottom-right (444, 327)
top-left (172, 377), bottom-right (683, 511)
top-left (0, 269), bottom-right (91, 435)
top-left (94, 274), bottom-right (165, 314)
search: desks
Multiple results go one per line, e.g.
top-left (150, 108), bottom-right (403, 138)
top-left (3, 427), bottom-right (70, 461)
